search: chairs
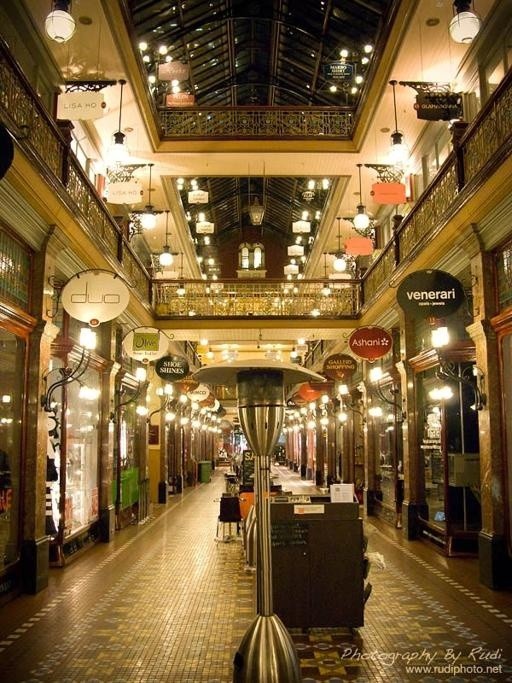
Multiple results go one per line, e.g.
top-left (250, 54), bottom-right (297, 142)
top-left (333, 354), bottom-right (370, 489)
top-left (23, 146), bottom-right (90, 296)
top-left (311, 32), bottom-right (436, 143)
top-left (214, 476), bottom-right (284, 540)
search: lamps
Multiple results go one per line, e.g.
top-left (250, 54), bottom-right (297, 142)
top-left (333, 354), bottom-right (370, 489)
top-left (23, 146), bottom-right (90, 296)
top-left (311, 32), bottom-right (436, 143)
top-left (320, 248), bottom-right (348, 299)
top-left (283, 382), bottom-right (362, 442)
top-left (66, 76), bottom-right (133, 163)
top-left (108, 366), bottom-right (150, 426)
top-left (147, 382), bottom-right (224, 436)
top-left (151, 251), bottom-right (188, 296)
top-left (350, 160), bottom-right (407, 230)
top-left (333, 213), bottom-right (373, 273)
top-left (426, 323), bottom-right (484, 411)
top-left (446, 0), bottom-right (483, 47)
top-left (247, 194), bottom-right (266, 225)
top-left (43, 0), bottom-right (80, 45)
top-left (119, 159), bottom-right (160, 229)
top-left (364, 367), bottom-right (404, 417)
top-left (382, 76), bottom-right (451, 163)
top-left (42, 327), bottom-right (97, 413)
top-left (133, 208), bottom-right (174, 266)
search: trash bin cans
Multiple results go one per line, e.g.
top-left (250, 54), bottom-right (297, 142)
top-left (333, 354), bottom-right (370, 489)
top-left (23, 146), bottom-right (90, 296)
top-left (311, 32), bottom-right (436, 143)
top-left (199, 461), bottom-right (212, 483)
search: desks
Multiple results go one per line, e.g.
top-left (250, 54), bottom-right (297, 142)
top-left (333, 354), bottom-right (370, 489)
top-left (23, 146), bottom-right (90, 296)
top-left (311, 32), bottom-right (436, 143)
top-left (267, 494), bottom-right (368, 633)
